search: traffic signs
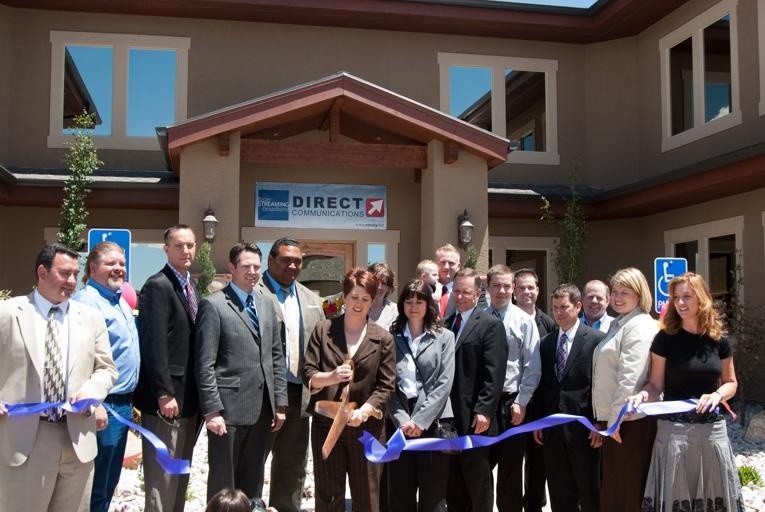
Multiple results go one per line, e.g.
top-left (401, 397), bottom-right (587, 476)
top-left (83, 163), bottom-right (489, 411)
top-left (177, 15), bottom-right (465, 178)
top-left (89, 229), bottom-right (130, 282)
top-left (655, 258), bottom-right (687, 314)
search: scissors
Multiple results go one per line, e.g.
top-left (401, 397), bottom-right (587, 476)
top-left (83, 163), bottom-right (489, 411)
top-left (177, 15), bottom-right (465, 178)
top-left (314, 359), bottom-right (358, 461)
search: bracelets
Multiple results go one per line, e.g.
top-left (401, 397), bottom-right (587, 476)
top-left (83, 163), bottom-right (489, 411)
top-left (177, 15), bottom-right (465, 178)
top-left (716, 389), bottom-right (725, 403)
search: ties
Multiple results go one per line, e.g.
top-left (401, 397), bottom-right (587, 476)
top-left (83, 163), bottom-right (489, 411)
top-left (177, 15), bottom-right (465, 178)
top-left (280, 288), bottom-right (300, 378)
top-left (556, 333), bottom-right (569, 381)
top-left (185, 281), bottom-right (198, 324)
top-left (43, 307), bottom-right (65, 423)
top-left (246, 295), bottom-right (260, 338)
top-left (439, 286), bottom-right (449, 317)
top-left (451, 313), bottom-right (462, 342)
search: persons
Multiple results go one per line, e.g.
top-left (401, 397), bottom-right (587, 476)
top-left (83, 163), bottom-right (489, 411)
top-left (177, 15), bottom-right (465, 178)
top-left (1, 243), bottom-right (117, 512)
top-left (533, 284), bottom-right (608, 512)
top-left (257, 238), bottom-right (327, 511)
top-left (513, 271), bottom-right (558, 510)
top-left (197, 243), bottom-right (286, 504)
top-left (573, 280), bottom-right (615, 333)
top-left (626, 272), bottom-right (744, 511)
top-left (484, 264), bottom-right (543, 511)
top-left (138, 224), bottom-right (203, 511)
top-left (441, 269), bottom-right (507, 510)
top-left (361, 262), bottom-right (400, 332)
top-left (385, 285), bottom-right (458, 510)
top-left (592, 267), bottom-right (656, 510)
top-left (413, 260), bottom-right (442, 324)
top-left (434, 246), bottom-right (463, 329)
top-left (304, 267), bottom-right (398, 512)
top-left (74, 242), bottom-right (139, 510)
